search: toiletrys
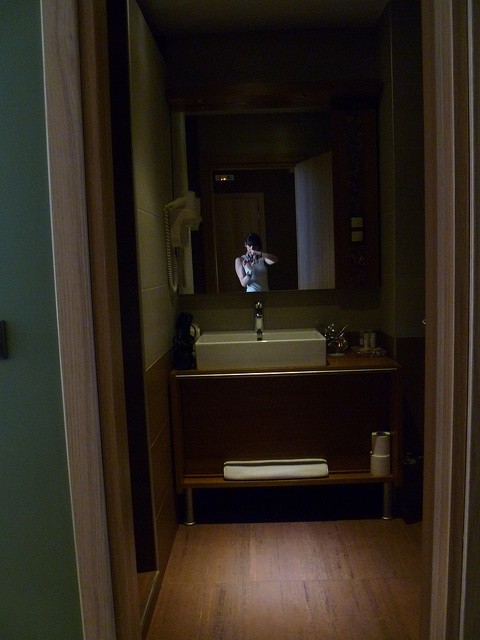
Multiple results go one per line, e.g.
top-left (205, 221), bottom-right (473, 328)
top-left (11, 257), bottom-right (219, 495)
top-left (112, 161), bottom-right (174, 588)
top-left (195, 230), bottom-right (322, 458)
top-left (358, 325), bottom-right (377, 348)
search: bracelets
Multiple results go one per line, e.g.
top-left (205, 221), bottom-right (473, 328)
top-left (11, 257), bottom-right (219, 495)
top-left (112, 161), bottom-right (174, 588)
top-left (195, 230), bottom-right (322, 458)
top-left (245, 271), bottom-right (252, 278)
top-left (260, 251), bottom-right (263, 257)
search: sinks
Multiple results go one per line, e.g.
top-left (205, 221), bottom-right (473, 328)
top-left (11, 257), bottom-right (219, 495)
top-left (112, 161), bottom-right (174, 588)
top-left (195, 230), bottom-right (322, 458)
top-left (194, 331), bottom-right (328, 368)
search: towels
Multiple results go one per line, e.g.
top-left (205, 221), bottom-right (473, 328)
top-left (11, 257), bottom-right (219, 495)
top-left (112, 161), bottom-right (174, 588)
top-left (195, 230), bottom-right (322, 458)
top-left (223, 457), bottom-right (329, 480)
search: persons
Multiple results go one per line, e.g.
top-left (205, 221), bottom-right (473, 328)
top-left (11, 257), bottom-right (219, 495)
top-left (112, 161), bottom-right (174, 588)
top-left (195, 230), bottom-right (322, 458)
top-left (234, 232), bottom-right (278, 292)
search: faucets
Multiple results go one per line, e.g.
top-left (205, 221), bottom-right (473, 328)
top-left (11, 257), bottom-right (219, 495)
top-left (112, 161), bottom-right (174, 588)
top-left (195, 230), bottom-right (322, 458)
top-left (253, 301), bottom-right (264, 332)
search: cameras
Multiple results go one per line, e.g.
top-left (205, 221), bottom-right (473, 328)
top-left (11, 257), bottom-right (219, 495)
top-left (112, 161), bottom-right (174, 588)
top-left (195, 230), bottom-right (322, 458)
top-left (247, 254), bottom-right (253, 262)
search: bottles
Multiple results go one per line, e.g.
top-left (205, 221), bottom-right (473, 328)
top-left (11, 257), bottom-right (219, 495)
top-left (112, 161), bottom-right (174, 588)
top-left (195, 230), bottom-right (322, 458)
top-left (370, 332), bottom-right (376, 348)
top-left (363, 333), bottom-right (370, 349)
top-left (359, 329), bottom-right (364, 347)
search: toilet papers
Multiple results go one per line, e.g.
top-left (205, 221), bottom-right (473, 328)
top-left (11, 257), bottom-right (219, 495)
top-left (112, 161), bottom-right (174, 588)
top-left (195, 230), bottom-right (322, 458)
top-left (371, 431), bottom-right (391, 454)
top-left (370, 454), bottom-right (391, 474)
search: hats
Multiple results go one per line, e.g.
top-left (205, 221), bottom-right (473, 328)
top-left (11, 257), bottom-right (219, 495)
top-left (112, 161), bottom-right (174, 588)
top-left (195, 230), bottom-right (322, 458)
top-left (245, 233), bottom-right (261, 245)
